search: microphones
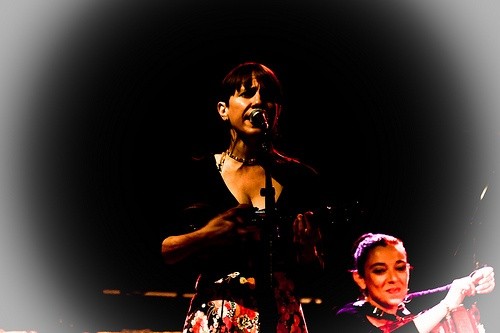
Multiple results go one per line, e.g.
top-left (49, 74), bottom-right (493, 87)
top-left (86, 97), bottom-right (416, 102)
top-left (250, 110), bottom-right (269, 130)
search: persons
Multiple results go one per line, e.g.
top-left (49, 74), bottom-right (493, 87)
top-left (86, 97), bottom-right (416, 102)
top-left (332, 233), bottom-right (495, 333)
top-left (128, 62), bottom-right (336, 333)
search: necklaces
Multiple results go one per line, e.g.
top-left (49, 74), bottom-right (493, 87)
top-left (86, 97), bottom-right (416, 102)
top-left (218, 150), bottom-right (259, 174)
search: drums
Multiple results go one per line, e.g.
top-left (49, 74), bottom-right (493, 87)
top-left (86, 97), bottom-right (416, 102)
top-left (379, 304), bottom-right (478, 333)
